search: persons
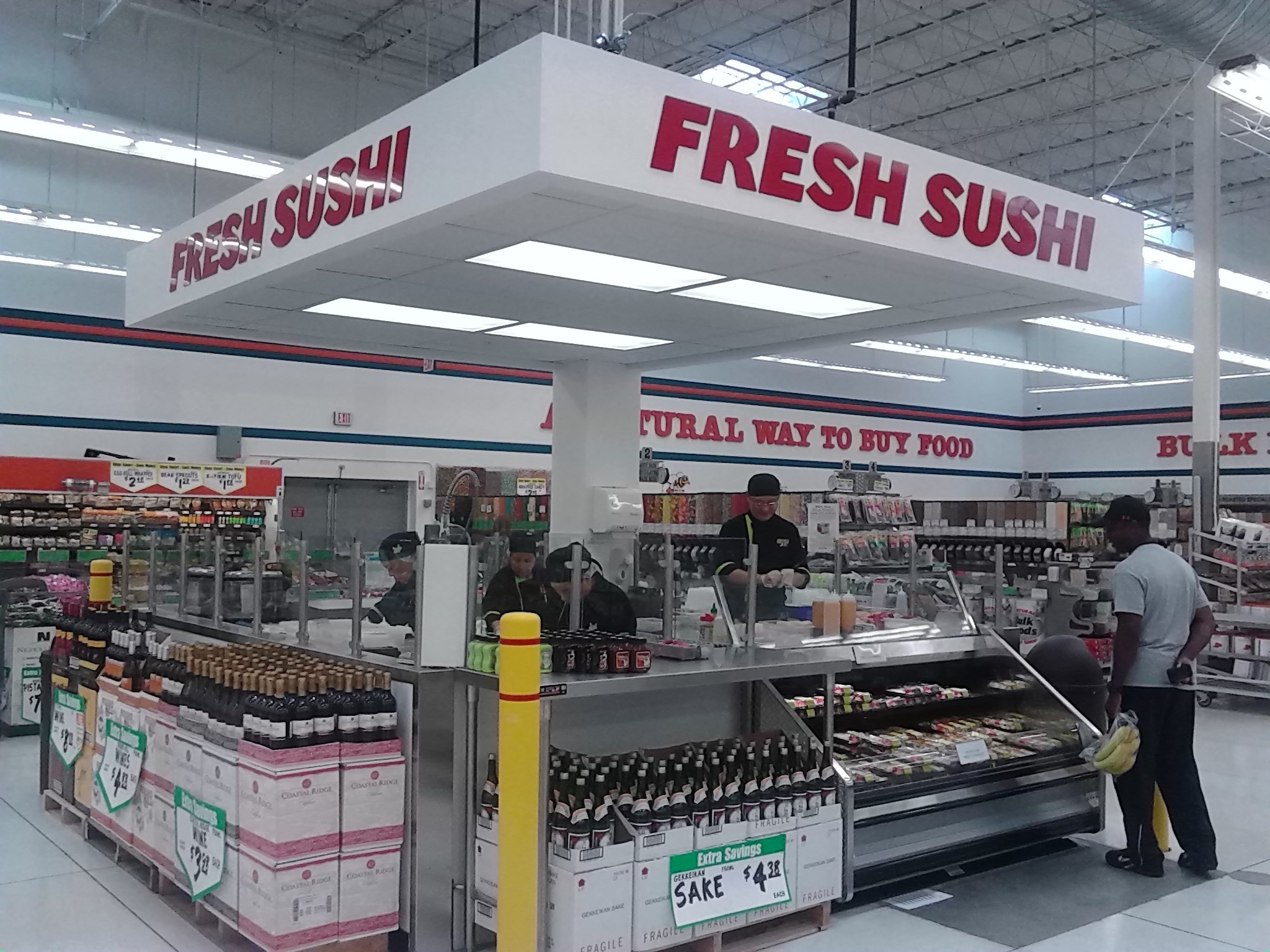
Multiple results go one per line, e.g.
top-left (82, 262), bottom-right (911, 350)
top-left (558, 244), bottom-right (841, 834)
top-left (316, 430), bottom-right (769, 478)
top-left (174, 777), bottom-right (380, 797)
top-left (1089, 496), bottom-right (1218, 879)
top-left (546, 541), bottom-right (638, 636)
top-left (713, 473), bottom-right (810, 626)
top-left (481, 532), bottom-right (562, 635)
top-left (361, 531), bottom-right (422, 631)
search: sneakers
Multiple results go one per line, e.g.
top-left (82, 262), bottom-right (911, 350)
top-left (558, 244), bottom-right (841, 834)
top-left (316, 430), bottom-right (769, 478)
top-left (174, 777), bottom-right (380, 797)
top-left (1178, 852), bottom-right (1214, 870)
top-left (1106, 848), bottom-right (1163, 876)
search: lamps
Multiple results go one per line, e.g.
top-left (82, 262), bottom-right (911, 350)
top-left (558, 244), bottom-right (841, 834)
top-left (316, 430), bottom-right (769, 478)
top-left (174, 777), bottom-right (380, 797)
top-left (0, 0), bottom-right (1270, 394)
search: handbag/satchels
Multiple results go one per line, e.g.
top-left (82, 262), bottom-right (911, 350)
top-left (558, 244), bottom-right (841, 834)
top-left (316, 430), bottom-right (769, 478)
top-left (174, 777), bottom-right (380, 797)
top-left (1079, 710), bottom-right (1141, 778)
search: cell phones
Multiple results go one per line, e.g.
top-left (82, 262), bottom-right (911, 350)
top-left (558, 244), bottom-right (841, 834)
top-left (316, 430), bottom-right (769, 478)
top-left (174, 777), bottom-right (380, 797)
top-left (1166, 664), bottom-right (1194, 685)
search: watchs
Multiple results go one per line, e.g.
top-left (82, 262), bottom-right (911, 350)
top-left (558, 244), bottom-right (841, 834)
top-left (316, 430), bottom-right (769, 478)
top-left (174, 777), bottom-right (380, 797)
top-left (1106, 681), bottom-right (1125, 695)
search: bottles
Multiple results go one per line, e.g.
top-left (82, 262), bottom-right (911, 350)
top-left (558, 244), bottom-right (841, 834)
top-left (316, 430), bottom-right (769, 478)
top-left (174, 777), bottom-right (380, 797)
top-left (699, 601), bottom-right (729, 644)
top-left (467, 627), bottom-right (652, 674)
top-left (895, 586), bottom-right (907, 616)
top-left (481, 733), bottom-right (837, 850)
top-left (812, 587), bottom-right (857, 638)
top-left (52, 597), bottom-right (397, 750)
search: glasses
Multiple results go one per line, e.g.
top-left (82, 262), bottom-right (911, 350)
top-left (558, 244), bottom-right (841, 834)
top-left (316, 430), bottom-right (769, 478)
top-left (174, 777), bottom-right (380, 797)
top-left (752, 499), bottom-right (778, 508)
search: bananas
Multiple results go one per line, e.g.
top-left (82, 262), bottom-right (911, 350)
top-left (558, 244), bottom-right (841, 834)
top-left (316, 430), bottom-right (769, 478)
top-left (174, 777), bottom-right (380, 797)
top-left (1092, 725), bottom-right (1141, 774)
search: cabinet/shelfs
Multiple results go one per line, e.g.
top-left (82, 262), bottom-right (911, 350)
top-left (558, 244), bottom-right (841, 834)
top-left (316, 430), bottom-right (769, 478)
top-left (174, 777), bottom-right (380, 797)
top-left (474, 521), bottom-right (811, 621)
top-left (912, 455), bottom-right (1270, 952)
top-left (0, 491), bottom-right (267, 616)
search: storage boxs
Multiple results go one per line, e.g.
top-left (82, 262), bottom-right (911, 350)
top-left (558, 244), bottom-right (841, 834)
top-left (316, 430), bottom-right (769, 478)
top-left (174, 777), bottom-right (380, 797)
top-left (474, 803), bottom-right (843, 952)
top-left (90, 656), bottom-right (405, 952)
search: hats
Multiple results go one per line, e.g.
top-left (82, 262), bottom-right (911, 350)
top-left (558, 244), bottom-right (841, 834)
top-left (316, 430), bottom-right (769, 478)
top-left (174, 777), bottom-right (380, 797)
top-left (1084, 495), bottom-right (1151, 528)
